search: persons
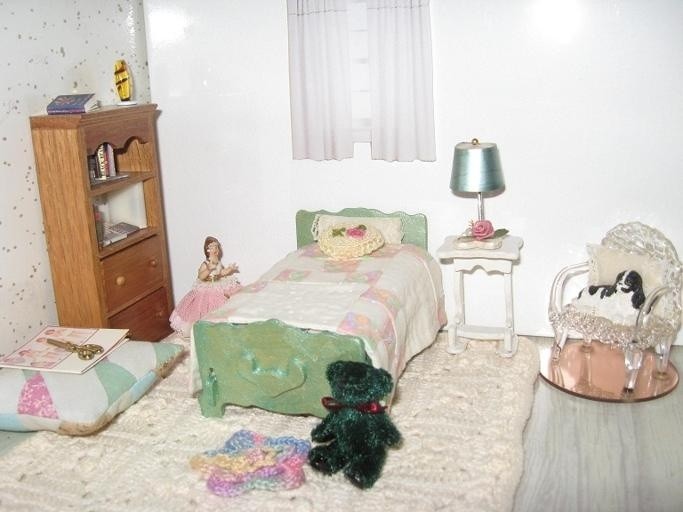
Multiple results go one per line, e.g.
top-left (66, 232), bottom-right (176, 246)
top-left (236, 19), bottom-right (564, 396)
top-left (167, 235), bottom-right (244, 339)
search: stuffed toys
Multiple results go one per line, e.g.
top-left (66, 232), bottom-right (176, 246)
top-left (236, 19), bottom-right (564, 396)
top-left (306, 360), bottom-right (403, 493)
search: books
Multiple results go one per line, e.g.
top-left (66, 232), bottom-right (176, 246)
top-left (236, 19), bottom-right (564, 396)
top-left (87, 148), bottom-right (102, 179)
top-left (45, 92), bottom-right (99, 114)
top-left (100, 220), bottom-right (127, 247)
top-left (112, 221), bottom-right (141, 234)
top-left (107, 143), bottom-right (117, 177)
top-left (97, 141), bottom-right (110, 180)
top-left (0, 324), bottom-right (132, 377)
